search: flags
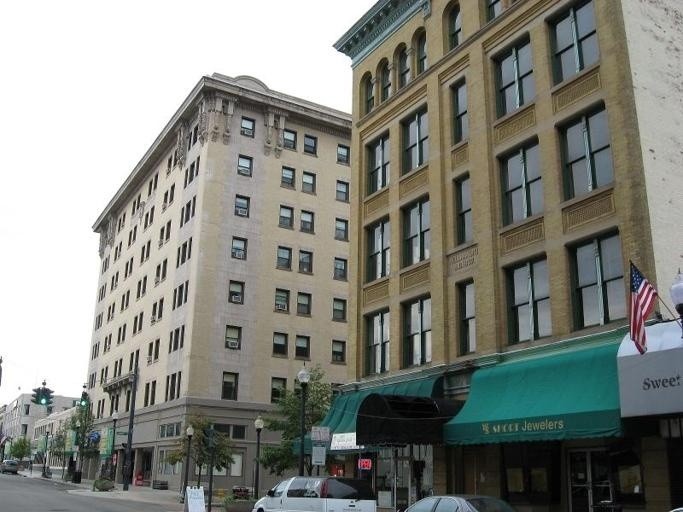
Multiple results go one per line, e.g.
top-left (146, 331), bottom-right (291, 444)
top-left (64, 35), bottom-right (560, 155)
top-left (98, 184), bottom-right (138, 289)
top-left (628, 261), bottom-right (657, 355)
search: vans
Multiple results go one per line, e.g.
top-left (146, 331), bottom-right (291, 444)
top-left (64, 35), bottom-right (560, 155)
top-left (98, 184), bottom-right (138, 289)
top-left (251, 476), bottom-right (376, 512)
top-left (0, 460), bottom-right (18, 474)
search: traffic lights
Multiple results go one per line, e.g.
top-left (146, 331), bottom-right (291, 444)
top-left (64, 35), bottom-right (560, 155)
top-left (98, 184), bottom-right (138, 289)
top-left (31, 387), bottom-right (54, 404)
top-left (80, 391), bottom-right (89, 408)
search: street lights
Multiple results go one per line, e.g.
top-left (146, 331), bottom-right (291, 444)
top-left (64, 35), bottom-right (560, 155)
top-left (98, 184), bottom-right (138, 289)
top-left (669, 266), bottom-right (683, 338)
top-left (110, 409), bottom-right (118, 487)
top-left (75, 419), bottom-right (81, 438)
top-left (45, 422), bottom-right (50, 476)
top-left (297, 359), bottom-right (311, 476)
top-left (254, 412), bottom-right (264, 499)
top-left (181, 422), bottom-right (194, 503)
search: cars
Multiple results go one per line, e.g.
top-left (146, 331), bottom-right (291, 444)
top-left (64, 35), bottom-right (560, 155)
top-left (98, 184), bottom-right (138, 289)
top-left (404, 494), bottom-right (518, 512)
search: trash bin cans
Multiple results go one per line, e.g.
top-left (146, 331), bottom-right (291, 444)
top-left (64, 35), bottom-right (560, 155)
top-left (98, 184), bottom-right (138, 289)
top-left (72, 472), bottom-right (82, 483)
